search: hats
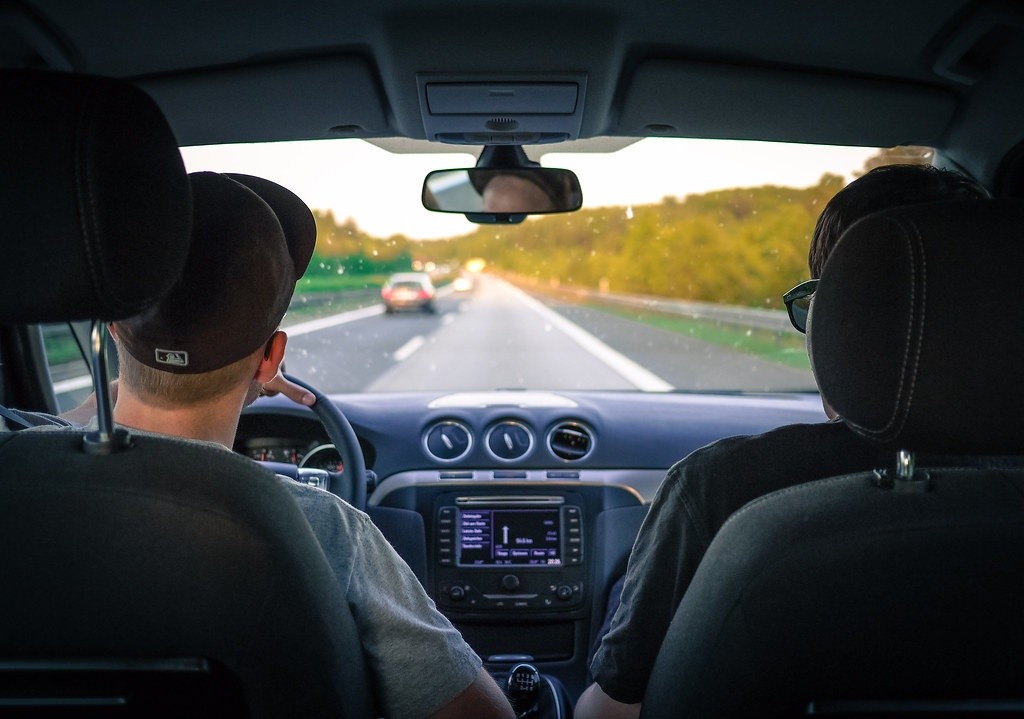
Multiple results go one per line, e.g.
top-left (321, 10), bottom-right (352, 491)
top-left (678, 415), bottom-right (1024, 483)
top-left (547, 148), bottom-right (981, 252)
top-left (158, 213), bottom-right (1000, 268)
top-left (467, 170), bottom-right (567, 213)
top-left (111, 171), bottom-right (318, 375)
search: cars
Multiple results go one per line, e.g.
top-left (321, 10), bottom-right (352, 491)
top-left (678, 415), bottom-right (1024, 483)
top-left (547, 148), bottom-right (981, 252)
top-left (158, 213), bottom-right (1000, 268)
top-left (379, 271), bottom-right (437, 314)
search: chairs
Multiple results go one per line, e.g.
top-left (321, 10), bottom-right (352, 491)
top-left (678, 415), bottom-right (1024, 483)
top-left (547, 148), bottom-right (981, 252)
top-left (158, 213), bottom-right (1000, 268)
top-left (637, 208), bottom-right (1024, 719)
top-left (1, 67), bottom-right (389, 719)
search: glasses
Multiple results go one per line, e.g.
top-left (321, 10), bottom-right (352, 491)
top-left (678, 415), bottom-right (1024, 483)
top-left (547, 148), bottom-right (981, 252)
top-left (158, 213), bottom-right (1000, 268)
top-left (783, 278), bottom-right (820, 335)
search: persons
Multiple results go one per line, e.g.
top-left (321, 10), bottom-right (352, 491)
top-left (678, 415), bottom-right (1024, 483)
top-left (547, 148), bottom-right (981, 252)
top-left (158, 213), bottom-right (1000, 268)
top-left (1, 172), bottom-right (519, 719)
top-left (482, 172), bottom-right (570, 210)
top-left (572, 164), bottom-right (991, 719)
